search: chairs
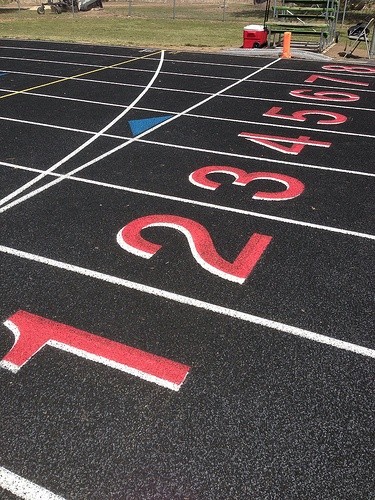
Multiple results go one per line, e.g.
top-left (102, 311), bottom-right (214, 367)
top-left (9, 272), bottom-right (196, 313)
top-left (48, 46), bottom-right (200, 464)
top-left (344, 17), bottom-right (375, 59)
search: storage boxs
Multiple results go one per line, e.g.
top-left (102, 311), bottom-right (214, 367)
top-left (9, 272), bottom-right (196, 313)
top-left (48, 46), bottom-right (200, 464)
top-left (242, 25), bottom-right (268, 48)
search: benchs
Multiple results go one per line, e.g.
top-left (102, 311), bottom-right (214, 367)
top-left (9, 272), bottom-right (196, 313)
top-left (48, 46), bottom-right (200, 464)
top-left (264, 0), bottom-right (341, 47)
